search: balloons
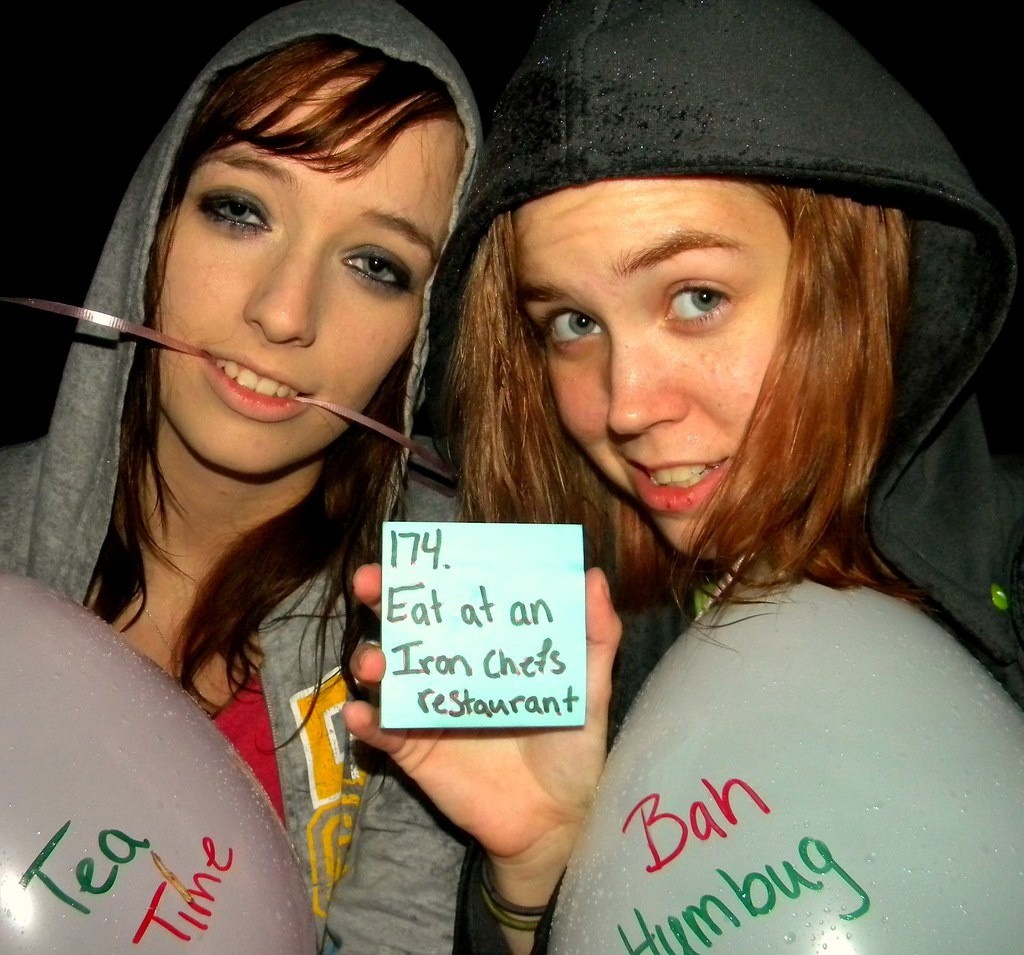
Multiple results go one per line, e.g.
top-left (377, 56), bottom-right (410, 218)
top-left (546, 579), bottom-right (1024, 953)
top-left (0, 573), bottom-right (321, 955)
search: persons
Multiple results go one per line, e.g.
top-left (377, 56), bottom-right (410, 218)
top-left (1, 1), bottom-right (483, 955)
top-left (346, 1), bottom-right (1024, 955)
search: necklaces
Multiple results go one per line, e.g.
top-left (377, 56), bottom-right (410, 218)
top-left (133, 583), bottom-right (205, 700)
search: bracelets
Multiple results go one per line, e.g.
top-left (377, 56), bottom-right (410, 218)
top-left (479, 861), bottom-right (549, 931)
